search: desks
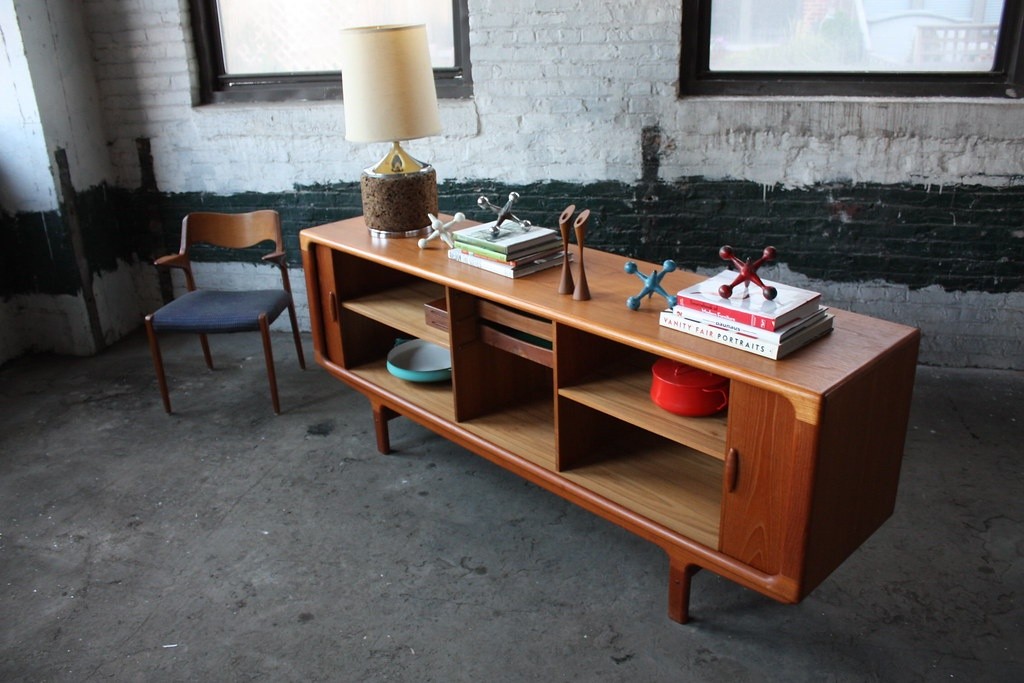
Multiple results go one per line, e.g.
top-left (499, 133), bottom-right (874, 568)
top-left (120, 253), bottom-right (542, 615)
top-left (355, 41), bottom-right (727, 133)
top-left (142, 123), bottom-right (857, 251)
top-left (299, 210), bottom-right (922, 624)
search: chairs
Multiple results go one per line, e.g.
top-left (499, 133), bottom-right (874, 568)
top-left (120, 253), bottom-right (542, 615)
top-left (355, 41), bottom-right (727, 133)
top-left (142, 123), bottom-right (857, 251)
top-left (145, 210), bottom-right (307, 413)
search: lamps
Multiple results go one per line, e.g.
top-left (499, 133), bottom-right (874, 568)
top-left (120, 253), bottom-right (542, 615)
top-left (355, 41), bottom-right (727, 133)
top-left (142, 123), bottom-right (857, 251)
top-left (337, 23), bottom-right (441, 238)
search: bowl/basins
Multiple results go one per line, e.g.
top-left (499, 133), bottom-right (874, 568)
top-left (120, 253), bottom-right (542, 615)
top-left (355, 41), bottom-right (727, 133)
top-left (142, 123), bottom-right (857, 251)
top-left (386, 338), bottom-right (453, 382)
top-left (650, 358), bottom-right (729, 417)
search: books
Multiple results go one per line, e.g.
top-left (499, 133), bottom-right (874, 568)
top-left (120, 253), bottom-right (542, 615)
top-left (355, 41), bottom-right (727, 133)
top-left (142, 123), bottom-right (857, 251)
top-left (659, 269), bottom-right (835, 361)
top-left (448, 218), bottom-right (574, 279)
